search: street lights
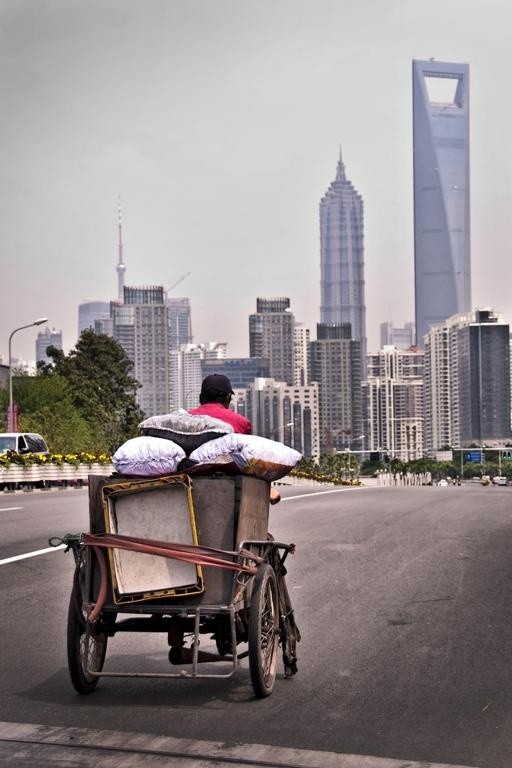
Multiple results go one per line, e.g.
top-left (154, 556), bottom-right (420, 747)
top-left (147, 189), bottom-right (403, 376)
top-left (270, 422), bottom-right (294, 439)
top-left (349, 435), bottom-right (366, 480)
top-left (9, 318), bottom-right (48, 433)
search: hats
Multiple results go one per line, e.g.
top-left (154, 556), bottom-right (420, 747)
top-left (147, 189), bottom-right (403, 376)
top-left (201, 374), bottom-right (235, 397)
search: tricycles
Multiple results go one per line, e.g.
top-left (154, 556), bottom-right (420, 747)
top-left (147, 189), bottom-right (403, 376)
top-left (49, 474), bottom-right (301, 696)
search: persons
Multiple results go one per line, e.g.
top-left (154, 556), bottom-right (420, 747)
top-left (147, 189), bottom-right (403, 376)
top-left (188, 374), bottom-right (253, 433)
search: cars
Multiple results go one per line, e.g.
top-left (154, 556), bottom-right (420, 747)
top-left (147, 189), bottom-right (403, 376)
top-left (0, 433), bottom-right (49, 490)
top-left (446, 475), bottom-right (507, 486)
